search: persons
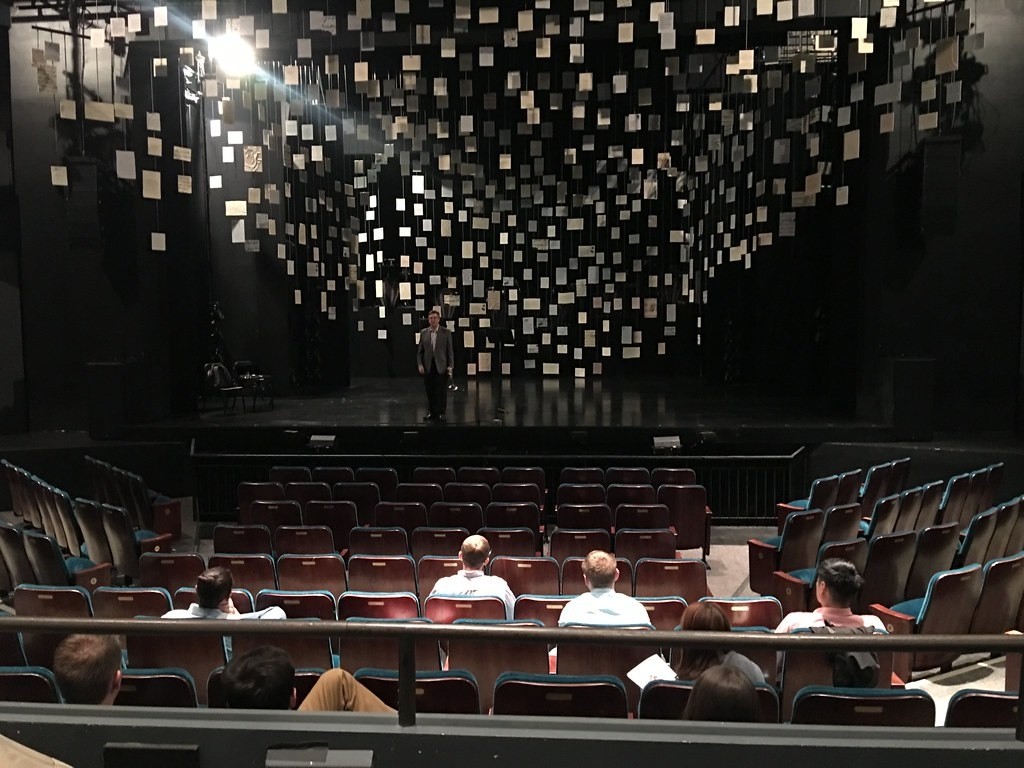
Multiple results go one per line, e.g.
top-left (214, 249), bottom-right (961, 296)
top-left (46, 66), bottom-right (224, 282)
top-left (50, 533), bottom-right (892, 723)
top-left (417, 310), bottom-right (455, 423)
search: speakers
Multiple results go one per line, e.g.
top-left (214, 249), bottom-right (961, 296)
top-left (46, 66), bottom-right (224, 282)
top-left (309, 434), bottom-right (340, 455)
top-left (652, 435), bottom-right (680, 456)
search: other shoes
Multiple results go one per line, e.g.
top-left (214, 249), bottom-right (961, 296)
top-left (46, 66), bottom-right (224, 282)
top-left (438, 413), bottom-right (447, 423)
top-left (423, 413), bottom-right (434, 421)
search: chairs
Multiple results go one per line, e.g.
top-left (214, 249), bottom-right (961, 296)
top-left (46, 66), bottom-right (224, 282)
top-left (202, 363), bottom-right (246, 416)
top-left (232, 360), bottom-right (274, 413)
top-left (0, 453), bottom-right (1024, 728)
